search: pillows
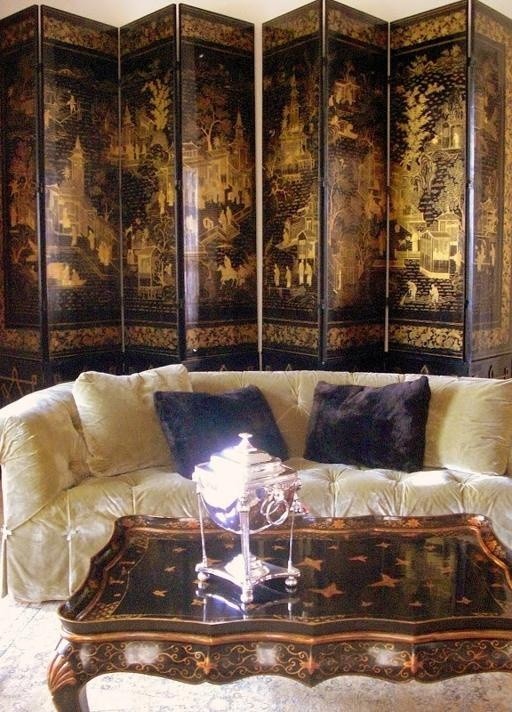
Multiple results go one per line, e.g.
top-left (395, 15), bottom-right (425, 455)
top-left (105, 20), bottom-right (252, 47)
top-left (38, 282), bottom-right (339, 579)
top-left (303, 376), bottom-right (432, 471)
top-left (75, 364), bottom-right (191, 479)
top-left (154, 385), bottom-right (288, 478)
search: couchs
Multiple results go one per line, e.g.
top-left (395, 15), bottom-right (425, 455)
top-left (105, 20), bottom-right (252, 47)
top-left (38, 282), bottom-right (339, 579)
top-left (0, 371), bottom-right (511, 614)
